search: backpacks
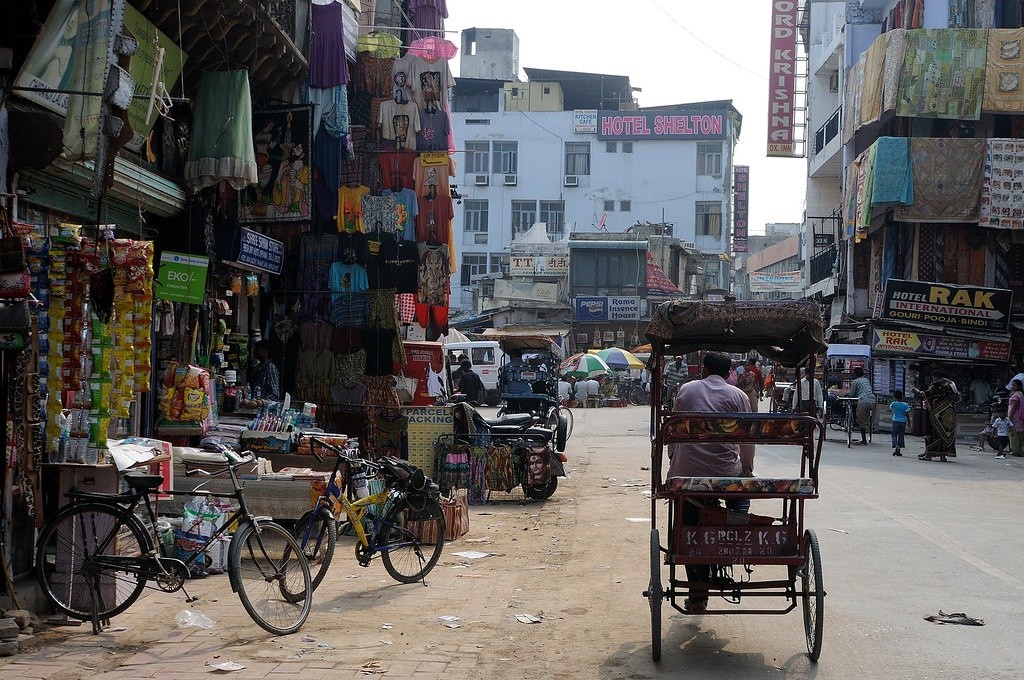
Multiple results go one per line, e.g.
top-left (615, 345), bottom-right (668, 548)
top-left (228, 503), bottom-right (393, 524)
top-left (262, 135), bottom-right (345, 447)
top-left (378, 454), bottom-right (432, 492)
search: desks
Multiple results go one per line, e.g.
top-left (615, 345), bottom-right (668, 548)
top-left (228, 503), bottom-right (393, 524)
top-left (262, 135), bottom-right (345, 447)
top-left (157, 474), bottom-right (343, 541)
top-left (956, 413), bottom-right (990, 440)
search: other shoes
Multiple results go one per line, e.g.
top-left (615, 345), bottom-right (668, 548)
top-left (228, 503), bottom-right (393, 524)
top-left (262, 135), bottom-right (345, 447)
top-left (940, 458), bottom-right (947, 462)
top-left (918, 452), bottom-right (925, 457)
top-left (893, 447), bottom-right (902, 456)
top-left (918, 456), bottom-right (932, 460)
top-left (856, 441), bottom-right (866, 445)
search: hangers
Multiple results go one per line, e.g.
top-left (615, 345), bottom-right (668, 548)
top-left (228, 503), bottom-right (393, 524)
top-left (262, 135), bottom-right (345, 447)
top-left (202, 50), bottom-right (249, 73)
top-left (378, 404), bottom-right (402, 419)
top-left (432, 435), bottom-right (523, 448)
top-left (253, 96), bottom-right (294, 107)
top-left (313, 314), bottom-right (323, 324)
top-left (343, 30), bottom-right (442, 250)
top-left (344, 401), bottom-right (358, 412)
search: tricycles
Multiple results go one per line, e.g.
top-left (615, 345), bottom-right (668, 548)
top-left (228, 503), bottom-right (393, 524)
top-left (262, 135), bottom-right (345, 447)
top-left (644, 298), bottom-right (834, 662)
top-left (823, 343), bottom-right (873, 448)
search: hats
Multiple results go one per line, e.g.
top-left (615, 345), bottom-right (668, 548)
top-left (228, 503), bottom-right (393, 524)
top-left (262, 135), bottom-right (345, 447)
top-left (675, 355), bottom-right (683, 359)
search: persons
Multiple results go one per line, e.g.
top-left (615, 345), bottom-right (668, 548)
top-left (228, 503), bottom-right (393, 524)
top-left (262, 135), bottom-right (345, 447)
top-left (1008, 379), bottom-right (1024, 457)
top-left (844, 368), bottom-right (876, 445)
top-left (660, 355), bottom-right (766, 412)
top-left (991, 409), bottom-right (1013, 460)
top-left (668, 352), bottom-right (756, 611)
top-left (453, 361), bottom-right (481, 408)
top-left (910, 370), bottom-right (960, 461)
top-left (503, 349), bottom-right (535, 397)
top-left (558, 376), bottom-right (615, 408)
top-left (248, 339), bottom-right (280, 402)
top-left (452, 354), bottom-right (469, 389)
top-left (887, 390), bottom-right (911, 456)
top-left (792, 368), bottom-right (823, 430)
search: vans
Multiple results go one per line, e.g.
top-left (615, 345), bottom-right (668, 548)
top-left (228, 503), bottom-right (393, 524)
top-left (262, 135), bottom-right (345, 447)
top-left (443, 340), bottom-right (509, 407)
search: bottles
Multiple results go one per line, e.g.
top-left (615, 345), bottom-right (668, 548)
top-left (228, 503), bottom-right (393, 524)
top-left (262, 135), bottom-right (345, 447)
top-left (254, 386), bottom-right (261, 400)
top-left (235, 387), bottom-right (242, 410)
top-left (244, 383), bottom-right (251, 401)
top-left (49, 411), bottom-right (67, 464)
top-left (217, 384), bottom-right (223, 415)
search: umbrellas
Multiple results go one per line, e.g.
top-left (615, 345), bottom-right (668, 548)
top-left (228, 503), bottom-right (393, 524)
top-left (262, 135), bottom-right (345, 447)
top-left (561, 354), bottom-right (612, 381)
top-left (596, 347), bottom-right (645, 384)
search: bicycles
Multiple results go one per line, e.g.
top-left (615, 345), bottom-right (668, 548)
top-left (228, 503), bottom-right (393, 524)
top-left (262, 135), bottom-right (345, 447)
top-left (276, 434), bottom-right (446, 604)
top-left (33, 446), bottom-right (314, 636)
top-left (613, 377), bottom-right (649, 407)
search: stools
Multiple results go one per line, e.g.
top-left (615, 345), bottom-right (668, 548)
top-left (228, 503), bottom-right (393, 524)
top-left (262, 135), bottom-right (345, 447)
top-left (606, 399), bottom-right (627, 407)
top-left (588, 399), bottom-right (600, 408)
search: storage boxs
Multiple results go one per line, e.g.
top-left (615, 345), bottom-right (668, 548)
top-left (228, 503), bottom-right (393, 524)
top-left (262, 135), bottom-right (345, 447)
top-left (297, 432), bottom-right (348, 457)
top-left (241, 430), bottom-right (295, 454)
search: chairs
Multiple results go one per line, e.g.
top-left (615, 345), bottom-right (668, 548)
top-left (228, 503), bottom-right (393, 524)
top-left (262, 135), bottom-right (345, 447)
top-left (451, 355), bottom-right (456, 362)
top-left (473, 352), bottom-right (485, 365)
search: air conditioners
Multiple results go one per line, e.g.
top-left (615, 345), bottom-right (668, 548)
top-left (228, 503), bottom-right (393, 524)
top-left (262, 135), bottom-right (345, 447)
top-left (830, 72), bottom-right (838, 93)
top-left (564, 175), bottom-right (578, 186)
top-left (503, 175), bottom-right (517, 186)
top-left (474, 175), bottom-right (489, 186)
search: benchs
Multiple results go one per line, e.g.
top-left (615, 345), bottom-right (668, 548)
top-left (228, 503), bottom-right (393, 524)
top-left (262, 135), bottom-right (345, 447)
top-left (827, 368), bottom-right (871, 397)
top-left (775, 381), bottom-right (794, 402)
top-left (660, 414), bottom-right (816, 495)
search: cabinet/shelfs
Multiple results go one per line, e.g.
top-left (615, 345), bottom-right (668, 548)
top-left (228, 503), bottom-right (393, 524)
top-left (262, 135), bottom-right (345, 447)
top-left (877, 395), bottom-right (923, 437)
top-left (34, 454), bottom-right (170, 614)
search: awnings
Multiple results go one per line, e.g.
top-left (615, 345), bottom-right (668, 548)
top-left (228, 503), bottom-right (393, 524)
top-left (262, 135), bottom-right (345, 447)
top-left (826, 344), bottom-right (872, 367)
